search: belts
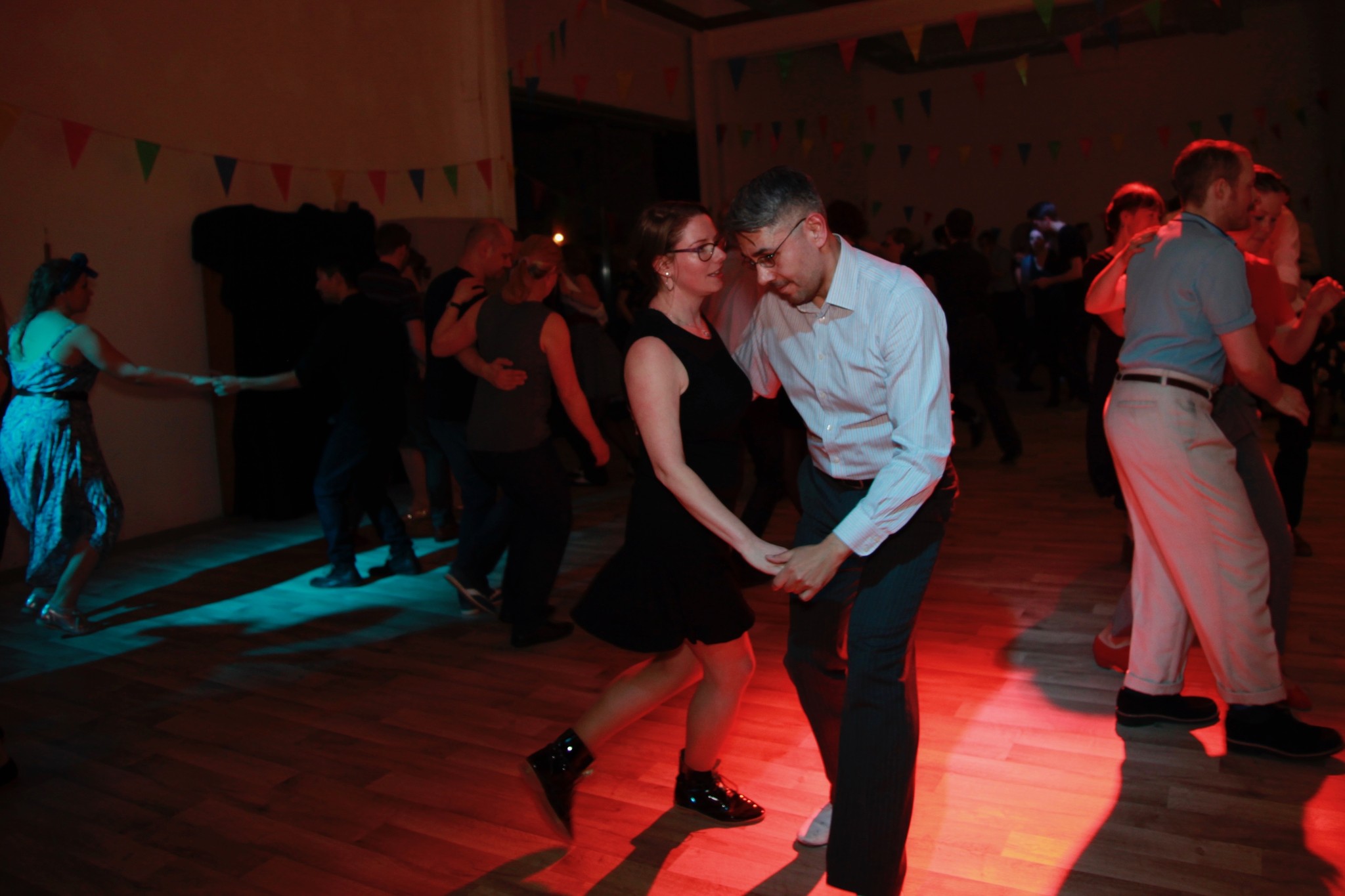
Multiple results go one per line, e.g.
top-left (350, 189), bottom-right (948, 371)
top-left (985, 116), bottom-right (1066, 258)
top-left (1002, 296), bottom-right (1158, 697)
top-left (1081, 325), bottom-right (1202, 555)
top-left (840, 479), bottom-right (871, 490)
top-left (1116, 373), bottom-right (1213, 402)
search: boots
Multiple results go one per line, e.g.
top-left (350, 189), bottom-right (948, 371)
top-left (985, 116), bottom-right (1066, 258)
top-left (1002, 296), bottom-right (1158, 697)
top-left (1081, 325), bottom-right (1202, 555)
top-left (517, 728), bottom-right (594, 840)
top-left (674, 747), bottom-right (766, 826)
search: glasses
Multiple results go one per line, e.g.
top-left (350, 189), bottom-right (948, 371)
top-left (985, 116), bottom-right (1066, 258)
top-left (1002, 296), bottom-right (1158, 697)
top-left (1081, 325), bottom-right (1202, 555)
top-left (667, 237), bottom-right (726, 262)
top-left (741, 218), bottom-right (807, 271)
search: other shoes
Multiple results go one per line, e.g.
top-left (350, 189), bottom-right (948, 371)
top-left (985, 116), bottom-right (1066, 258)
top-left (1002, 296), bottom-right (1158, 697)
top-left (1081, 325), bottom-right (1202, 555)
top-left (401, 508), bottom-right (430, 522)
top-left (456, 505), bottom-right (465, 513)
top-left (1292, 533), bottom-right (1313, 557)
top-left (445, 566), bottom-right (497, 615)
top-left (310, 566), bottom-right (362, 589)
top-left (797, 802), bottom-right (832, 847)
top-left (1223, 704), bottom-right (1345, 758)
top-left (460, 587), bottom-right (502, 615)
top-left (1115, 687), bottom-right (1219, 726)
top-left (968, 414), bottom-right (986, 448)
top-left (1093, 624), bottom-right (1130, 673)
top-left (511, 622), bottom-right (574, 647)
top-left (544, 604), bottom-right (557, 618)
top-left (368, 553), bottom-right (422, 579)
top-left (433, 520), bottom-right (459, 541)
top-left (21, 590), bottom-right (49, 618)
top-left (38, 603), bottom-right (91, 635)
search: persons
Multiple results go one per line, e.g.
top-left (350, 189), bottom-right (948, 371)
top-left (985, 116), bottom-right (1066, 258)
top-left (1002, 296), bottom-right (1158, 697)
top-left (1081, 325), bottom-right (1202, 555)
top-left (724, 168), bottom-right (960, 896)
top-left (1084, 139), bottom-right (1345, 755)
top-left (730, 395), bottom-right (807, 587)
top-left (524, 201), bottom-right (790, 837)
top-left (881, 200), bottom-right (1087, 464)
top-left (421, 217), bottom-right (642, 645)
top-left (221, 223), bottom-right (458, 585)
top-left (0, 258), bottom-right (221, 635)
